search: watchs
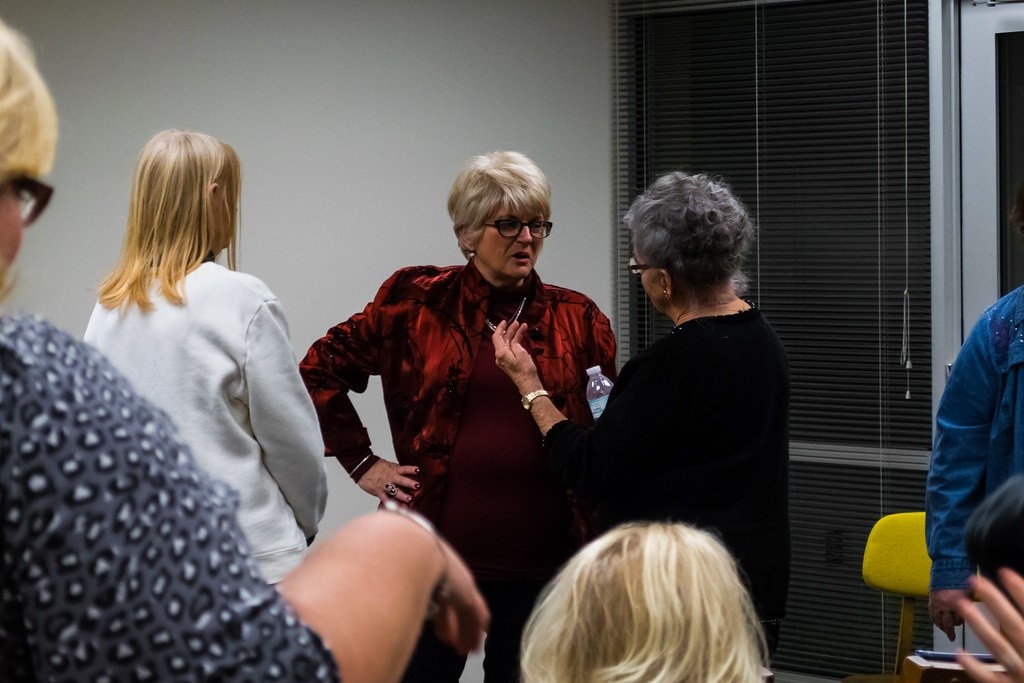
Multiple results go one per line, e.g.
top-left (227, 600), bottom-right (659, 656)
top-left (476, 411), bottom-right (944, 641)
top-left (521, 389), bottom-right (551, 410)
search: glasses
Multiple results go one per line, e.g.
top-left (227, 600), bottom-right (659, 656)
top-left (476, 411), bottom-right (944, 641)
top-left (627, 257), bottom-right (665, 274)
top-left (14, 175), bottom-right (54, 225)
top-left (483, 220), bottom-right (553, 239)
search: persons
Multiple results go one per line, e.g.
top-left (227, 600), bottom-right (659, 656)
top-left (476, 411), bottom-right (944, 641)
top-left (0, 18), bottom-right (492, 682)
top-left (492, 173), bottom-right (789, 618)
top-left (521, 520), bottom-right (773, 683)
top-left (297, 153), bottom-right (619, 683)
top-left (81, 129), bottom-right (328, 593)
top-left (924, 286), bottom-right (1024, 683)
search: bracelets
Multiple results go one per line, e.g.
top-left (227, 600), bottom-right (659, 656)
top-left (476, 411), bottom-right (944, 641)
top-left (382, 500), bottom-right (453, 600)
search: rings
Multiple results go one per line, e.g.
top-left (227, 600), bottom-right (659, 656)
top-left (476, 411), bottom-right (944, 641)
top-left (384, 483), bottom-right (400, 495)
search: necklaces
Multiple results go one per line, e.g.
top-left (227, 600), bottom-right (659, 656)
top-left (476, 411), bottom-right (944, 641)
top-left (676, 300), bottom-right (731, 322)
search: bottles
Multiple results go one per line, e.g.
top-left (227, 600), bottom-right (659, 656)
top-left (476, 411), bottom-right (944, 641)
top-left (585, 365), bottom-right (615, 422)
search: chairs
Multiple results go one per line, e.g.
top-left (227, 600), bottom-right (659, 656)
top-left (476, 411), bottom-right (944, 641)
top-left (843, 512), bottom-right (982, 683)
